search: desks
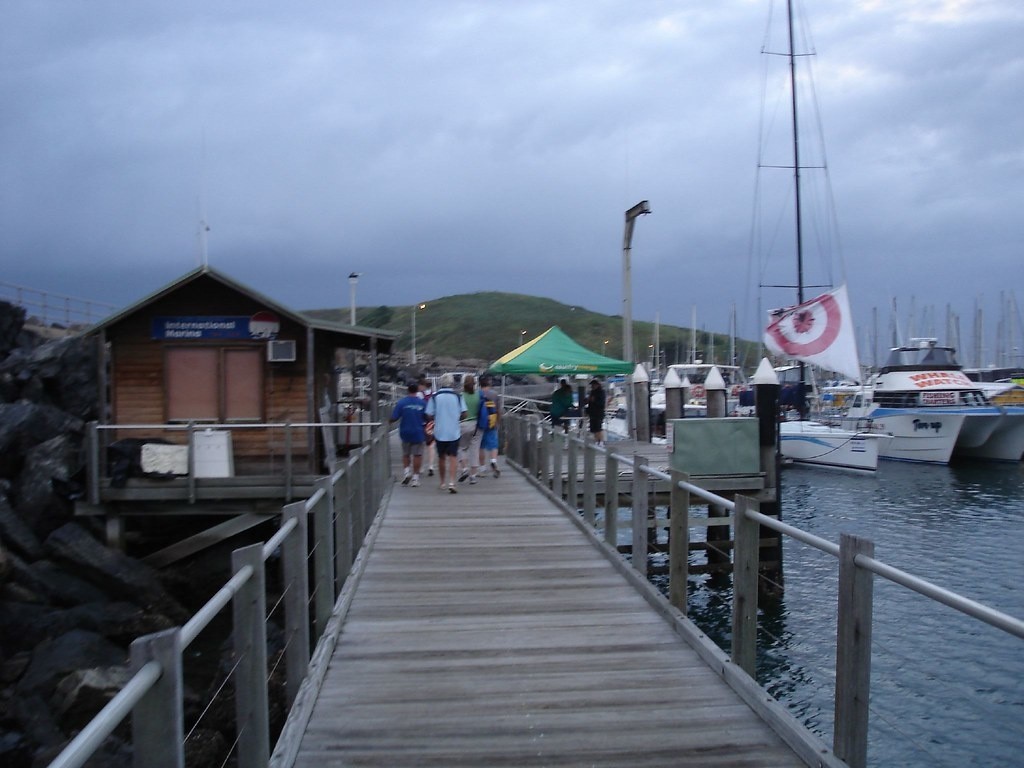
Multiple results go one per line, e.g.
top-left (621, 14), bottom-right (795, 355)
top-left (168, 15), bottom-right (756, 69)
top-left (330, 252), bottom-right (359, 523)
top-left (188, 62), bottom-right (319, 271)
top-left (560, 416), bottom-right (588, 434)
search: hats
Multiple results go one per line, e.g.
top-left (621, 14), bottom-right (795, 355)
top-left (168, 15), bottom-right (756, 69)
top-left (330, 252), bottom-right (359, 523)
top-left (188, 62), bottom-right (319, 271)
top-left (588, 380), bottom-right (598, 386)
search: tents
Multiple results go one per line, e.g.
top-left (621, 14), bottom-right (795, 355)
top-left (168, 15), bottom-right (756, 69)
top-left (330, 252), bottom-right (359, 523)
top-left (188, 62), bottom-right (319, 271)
top-left (489, 325), bottom-right (637, 442)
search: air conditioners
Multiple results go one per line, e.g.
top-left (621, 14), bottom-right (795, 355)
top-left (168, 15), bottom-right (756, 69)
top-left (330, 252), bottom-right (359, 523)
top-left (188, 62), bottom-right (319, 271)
top-left (266, 341), bottom-right (296, 361)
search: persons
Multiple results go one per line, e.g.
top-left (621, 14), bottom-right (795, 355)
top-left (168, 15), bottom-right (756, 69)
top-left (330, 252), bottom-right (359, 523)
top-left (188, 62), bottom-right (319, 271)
top-left (551, 378), bottom-right (605, 449)
top-left (390, 373), bottom-right (501, 493)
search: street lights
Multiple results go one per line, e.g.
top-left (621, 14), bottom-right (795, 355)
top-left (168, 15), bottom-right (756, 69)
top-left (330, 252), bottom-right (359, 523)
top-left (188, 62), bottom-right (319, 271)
top-left (602, 339), bottom-right (609, 358)
top-left (519, 328), bottom-right (526, 347)
top-left (350, 271), bottom-right (361, 325)
top-left (410, 302), bottom-right (427, 364)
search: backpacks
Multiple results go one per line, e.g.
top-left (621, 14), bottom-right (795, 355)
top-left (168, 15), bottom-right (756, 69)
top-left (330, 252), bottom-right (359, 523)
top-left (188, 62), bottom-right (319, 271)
top-left (477, 390), bottom-right (497, 430)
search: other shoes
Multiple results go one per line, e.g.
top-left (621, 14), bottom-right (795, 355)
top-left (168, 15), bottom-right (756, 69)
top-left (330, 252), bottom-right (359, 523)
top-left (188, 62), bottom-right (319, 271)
top-left (477, 470), bottom-right (486, 477)
top-left (428, 469), bottom-right (433, 476)
top-left (402, 472), bottom-right (411, 484)
top-left (448, 484), bottom-right (456, 493)
top-left (470, 477), bottom-right (476, 484)
top-left (441, 484), bottom-right (446, 490)
top-left (491, 461), bottom-right (500, 478)
top-left (411, 479), bottom-right (420, 487)
top-left (458, 470), bottom-right (468, 482)
top-left (594, 442), bottom-right (605, 448)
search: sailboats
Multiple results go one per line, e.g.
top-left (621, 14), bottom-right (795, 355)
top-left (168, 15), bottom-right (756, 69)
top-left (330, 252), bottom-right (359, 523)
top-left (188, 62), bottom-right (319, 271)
top-left (588, 0), bottom-right (1024, 477)
